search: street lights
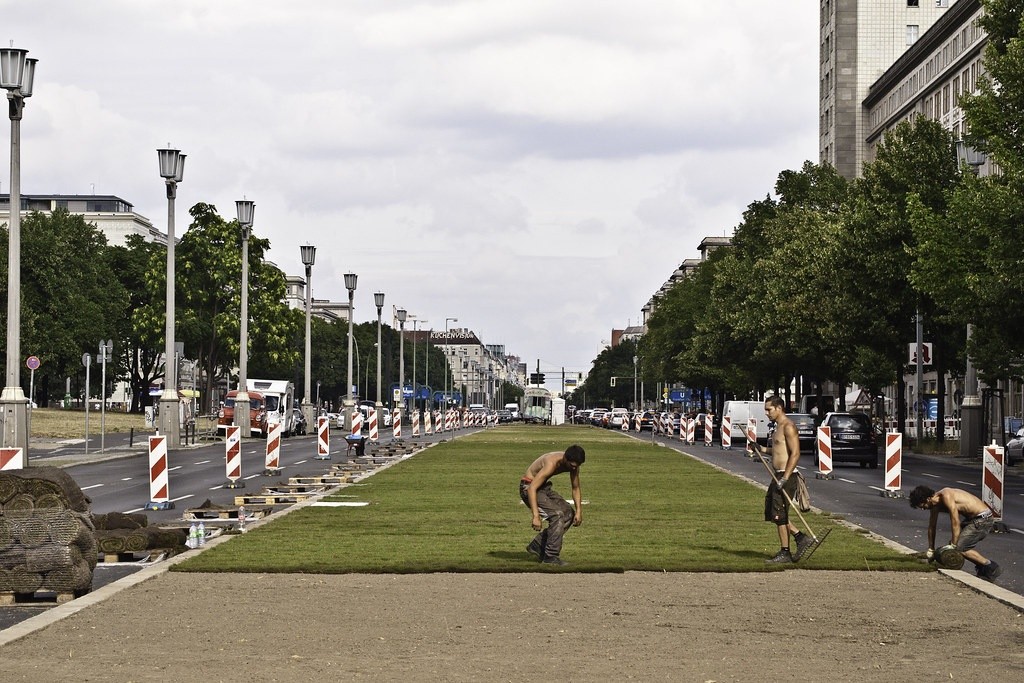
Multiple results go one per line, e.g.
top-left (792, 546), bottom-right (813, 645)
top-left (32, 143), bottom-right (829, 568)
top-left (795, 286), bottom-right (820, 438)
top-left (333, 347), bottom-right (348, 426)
top-left (412, 317), bottom-right (458, 423)
top-left (342, 269), bottom-right (358, 430)
top-left (233, 193), bottom-right (257, 440)
top-left (299, 240), bottom-right (317, 435)
top-left (373, 290), bottom-right (387, 429)
top-left (1, 39), bottom-right (39, 467)
top-left (396, 307), bottom-right (407, 427)
top-left (155, 143), bottom-right (187, 448)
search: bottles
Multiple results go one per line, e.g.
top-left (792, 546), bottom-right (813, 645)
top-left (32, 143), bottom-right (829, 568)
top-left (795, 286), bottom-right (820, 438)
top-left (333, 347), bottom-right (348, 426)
top-left (386, 460), bottom-right (389, 468)
top-left (750, 452), bottom-right (754, 463)
top-left (189, 521), bottom-right (206, 548)
top-left (238, 505), bottom-right (246, 529)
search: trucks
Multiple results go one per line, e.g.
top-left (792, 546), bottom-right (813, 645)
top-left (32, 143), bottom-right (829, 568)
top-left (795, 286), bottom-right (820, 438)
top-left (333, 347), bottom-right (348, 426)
top-left (237, 378), bottom-right (294, 438)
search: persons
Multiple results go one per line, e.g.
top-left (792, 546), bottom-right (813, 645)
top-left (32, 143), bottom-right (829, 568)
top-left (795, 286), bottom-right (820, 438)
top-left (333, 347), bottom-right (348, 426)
top-left (747, 396), bottom-right (811, 563)
top-left (909, 486), bottom-right (1002, 583)
top-left (520, 444), bottom-right (586, 566)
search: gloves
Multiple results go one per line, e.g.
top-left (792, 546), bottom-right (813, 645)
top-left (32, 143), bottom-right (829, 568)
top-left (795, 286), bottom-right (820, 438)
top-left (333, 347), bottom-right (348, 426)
top-left (942, 541), bottom-right (957, 550)
top-left (777, 477), bottom-right (790, 493)
top-left (926, 549), bottom-right (935, 558)
top-left (747, 441), bottom-right (761, 452)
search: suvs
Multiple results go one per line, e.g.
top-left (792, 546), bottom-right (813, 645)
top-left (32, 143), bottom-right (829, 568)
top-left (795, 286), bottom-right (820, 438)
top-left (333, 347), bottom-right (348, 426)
top-left (812, 411), bottom-right (878, 470)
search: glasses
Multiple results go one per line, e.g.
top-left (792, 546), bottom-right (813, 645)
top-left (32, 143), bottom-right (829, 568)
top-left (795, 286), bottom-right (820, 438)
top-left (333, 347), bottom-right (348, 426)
top-left (921, 503), bottom-right (927, 511)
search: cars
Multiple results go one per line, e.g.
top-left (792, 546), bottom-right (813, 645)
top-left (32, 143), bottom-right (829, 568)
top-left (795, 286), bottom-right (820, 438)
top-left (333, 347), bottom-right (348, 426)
top-left (1005, 424), bottom-right (1024, 467)
top-left (766, 413), bottom-right (822, 451)
top-left (217, 389), bottom-right (269, 440)
top-left (569, 407), bottom-right (720, 439)
top-left (466, 404), bottom-right (514, 423)
top-left (293, 400), bottom-right (393, 435)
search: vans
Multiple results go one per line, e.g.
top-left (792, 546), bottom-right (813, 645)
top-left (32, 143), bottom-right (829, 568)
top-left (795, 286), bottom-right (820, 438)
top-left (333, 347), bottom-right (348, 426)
top-left (720, 400), bottom-right (770, 439)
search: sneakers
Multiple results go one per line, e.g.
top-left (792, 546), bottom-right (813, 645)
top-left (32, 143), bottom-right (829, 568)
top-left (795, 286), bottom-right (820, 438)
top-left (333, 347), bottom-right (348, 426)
top-left (976, 559), bottom-right (1000, 583)
top-left (541, 556), bottom-right (568, 566)
top-left (793, 535), bottom-right (816, 563)
top-left (527, 540), bottom-right (545, 558)
top-left (764, 551), bottom-right (793, 564)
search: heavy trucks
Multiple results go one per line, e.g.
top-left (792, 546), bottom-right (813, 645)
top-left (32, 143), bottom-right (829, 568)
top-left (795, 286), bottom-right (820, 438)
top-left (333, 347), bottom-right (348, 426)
top-left (390, 383), bottom-right (461, 425)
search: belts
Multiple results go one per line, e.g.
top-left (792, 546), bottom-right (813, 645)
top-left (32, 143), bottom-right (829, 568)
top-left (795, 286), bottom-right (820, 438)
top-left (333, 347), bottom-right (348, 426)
top-left (970, 515), bottom-right (982, 521)
top-left (520, 480), bottom-right (531, 485)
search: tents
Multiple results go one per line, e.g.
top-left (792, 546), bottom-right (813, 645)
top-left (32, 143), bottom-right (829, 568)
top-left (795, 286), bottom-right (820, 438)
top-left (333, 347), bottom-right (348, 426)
top-left (834, 390), bottom-right (892, 418)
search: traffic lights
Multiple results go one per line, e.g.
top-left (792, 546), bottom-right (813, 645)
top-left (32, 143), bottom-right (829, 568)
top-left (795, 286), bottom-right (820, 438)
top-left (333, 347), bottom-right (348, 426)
top-left (579, 373), bottom-right (581, 381)
top-left (611, 377), bottom-right (615, 387)
